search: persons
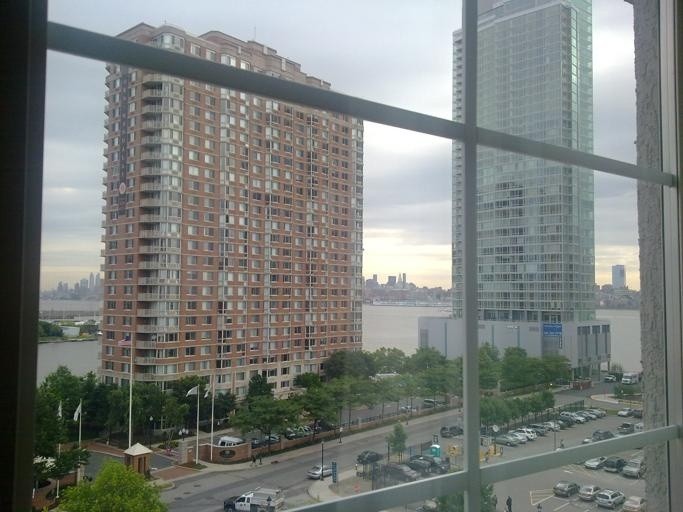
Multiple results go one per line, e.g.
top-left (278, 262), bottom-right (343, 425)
top-left (491, 494), bottom-right (497, 510)
top-left (249, 449), bottom-right (258, 467)
top-left (536, 503), bottom-right (542, 512)
top-left (505, 496), bottom-right (513, 512)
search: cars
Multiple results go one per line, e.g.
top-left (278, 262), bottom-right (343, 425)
top-left (617, 408), bottom-right (643, 418)
top-left (285, 422), bottom-right (321, 440)
top-left (579, 485), bottom-right (600, 500)
top-left (492, 407), bottom-right (607, 446)
top-left (401, 405), bottom-right (416, 412)
top-left (358, 449), bottom-right (384, 464)
top-left (622, 496), bottom-right (647, 512)
top-left (604, 374), bottom-right (616, 383)
top-left (584, 453), bottom-right (608, 470)
top-left (246, 434), bottom-right (281, 449)
top-left (307, 463), bottom-right (332, 479)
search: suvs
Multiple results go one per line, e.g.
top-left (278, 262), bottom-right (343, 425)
top-left (380, 455), bottom-right (450, 483)
top-left (594, 490), bottom-right (626, 508)
top-left (553, 480), bottom-right (580, 497)
top-left (603, 455), bottom-right (626, 473)
top-left (622, 459), bottom-right (647, 479)
top-left (441, 425), bottom-right (463, 437)
top-left (592, 428), bottom-right (614, 442)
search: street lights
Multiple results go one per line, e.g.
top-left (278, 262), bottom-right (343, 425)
top-left (179, 426), bottom-right (189, 441)
top-left (149, 415), bottom-right (154, 449)
top-left (320, 439), bottom-right (324, 480)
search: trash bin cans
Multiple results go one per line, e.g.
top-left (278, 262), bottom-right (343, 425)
top-left (430, 445), bottom-right (440, 457)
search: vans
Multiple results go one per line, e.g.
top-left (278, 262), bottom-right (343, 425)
top-left (424, 399), bottom-right (439, 408)
top-left (218, 436), bottom-right (243, 446)
top-left (633, 423), bottom-right (644, 433)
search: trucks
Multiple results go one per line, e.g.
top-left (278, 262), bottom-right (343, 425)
top-left (622, 372), bottom-right (640, 384)
top-left (224, 487), bottom-right (285, 512)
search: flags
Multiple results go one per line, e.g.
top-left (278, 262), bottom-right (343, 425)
top-left (117, 336), bottom-right (132, 347)
top-left (203, 383), bottom-right (211, 400)
top-left (185, 386), bottom-right (198, 397)
top-left (55, 402), bottom-right (63, 421)
top-left (72, 401), bottom-right (81, 421)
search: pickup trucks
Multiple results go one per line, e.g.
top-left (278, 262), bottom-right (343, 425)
top-left (617, 422), bottom-right (634, 433)
